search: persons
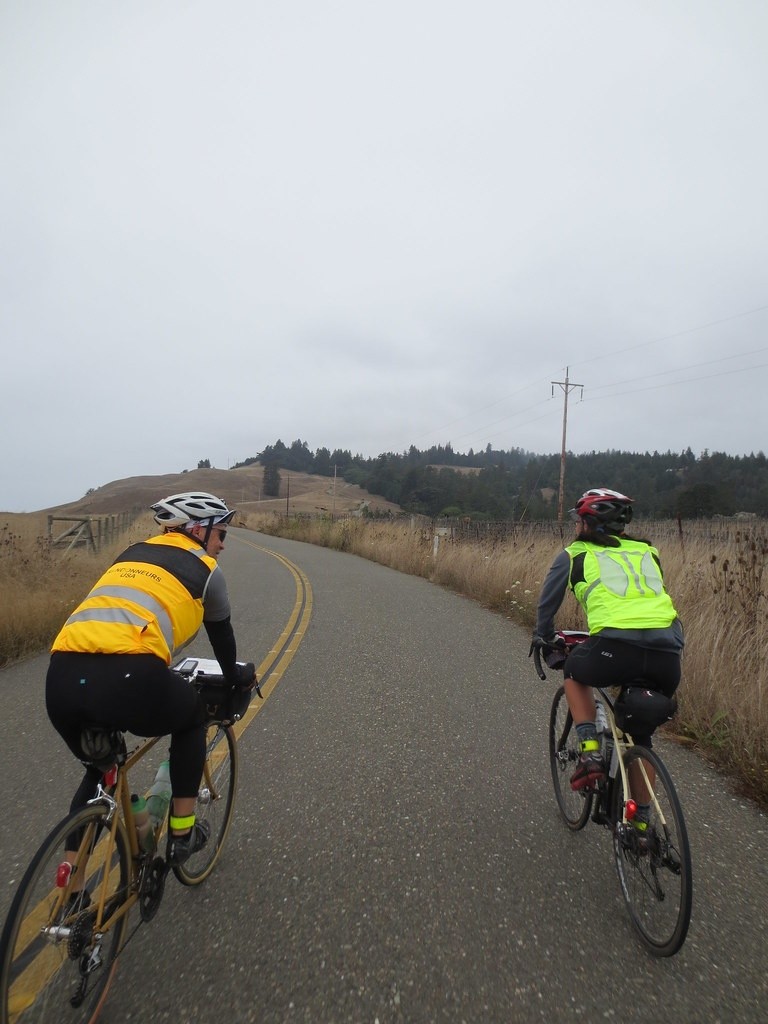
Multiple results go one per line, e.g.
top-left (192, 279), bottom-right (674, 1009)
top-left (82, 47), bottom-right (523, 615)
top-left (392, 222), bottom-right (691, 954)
top-left (45, 492), bottom-right (256, 926)
top-left (536, 488), bottom-right (684, 857)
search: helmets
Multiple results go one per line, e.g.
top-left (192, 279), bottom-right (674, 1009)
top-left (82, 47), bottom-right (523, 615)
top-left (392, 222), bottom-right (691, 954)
top-left (574, 488), bottom-right (636, 519)
top-left (150, 492), bottom-right (230, 532)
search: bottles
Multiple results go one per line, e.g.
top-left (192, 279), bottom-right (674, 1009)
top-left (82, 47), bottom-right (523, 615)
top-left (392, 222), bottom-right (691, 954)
top-left (600, 728), bottom-right (614, 764)
top-left (146, 760), bottom-right (172, 830)
top-left (595, 699), bottom-right (608, 735)
top-left (131, 793), bottom-right (153, 852)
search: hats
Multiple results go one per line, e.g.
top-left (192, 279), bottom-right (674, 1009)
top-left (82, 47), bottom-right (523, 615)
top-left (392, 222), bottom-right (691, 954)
top-left (168, 509), bottom-right (239, 530)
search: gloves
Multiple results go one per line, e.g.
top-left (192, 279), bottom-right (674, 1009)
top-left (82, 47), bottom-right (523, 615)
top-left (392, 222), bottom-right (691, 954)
top-left (235, 664), bottom-right (252, 687)
top-left (542, 634), bottom-right (568, 655)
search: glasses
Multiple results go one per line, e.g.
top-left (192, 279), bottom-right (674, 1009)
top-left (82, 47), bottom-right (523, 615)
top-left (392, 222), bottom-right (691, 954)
top-left (575, 520), bottom-right (582, 526)
top-left (211, 527), bottom-right (228, 543)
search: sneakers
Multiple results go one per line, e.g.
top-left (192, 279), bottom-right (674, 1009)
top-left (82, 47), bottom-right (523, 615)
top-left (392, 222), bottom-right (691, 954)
top-left (60, 889), bottom-right (94, 928)
top-left (165, 819), bottom-right (211, 867)
top-left (569, 739), bottom-right (606, 791)
top-left (627, 819), bottom-right (649, 838)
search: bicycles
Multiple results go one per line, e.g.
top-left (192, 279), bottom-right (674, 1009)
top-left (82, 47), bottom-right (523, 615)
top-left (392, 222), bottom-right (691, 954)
top-left (527, 629), bottom-right (695, 958)
top-left (0, 659), bottom-right (266, 1024)
